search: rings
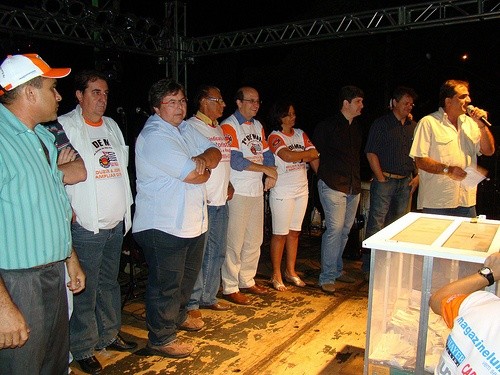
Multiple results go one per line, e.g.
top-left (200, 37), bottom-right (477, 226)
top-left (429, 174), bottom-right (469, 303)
top-left (77, 281), bottom-right (79, 283)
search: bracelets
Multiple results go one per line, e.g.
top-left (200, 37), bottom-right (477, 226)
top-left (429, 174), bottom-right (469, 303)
top-left (205, 168), bottom-right (211, 174)
top-left (300, 159), bottom-right (303, 163)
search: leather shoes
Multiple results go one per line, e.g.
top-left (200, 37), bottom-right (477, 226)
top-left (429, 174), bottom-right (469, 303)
top-left (107, 334), bottom-right (138, 350)
top-left (240, 284), bottom-right (267, 294)
top-left (76, 355), bottom-right (102, 374)
top-left (225, 292), bottom-right (251, 305)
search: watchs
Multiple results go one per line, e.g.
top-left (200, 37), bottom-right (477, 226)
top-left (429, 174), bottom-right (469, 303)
top-left (478, 267), bottom-right (494, 286)
top-left (443, 165), bottom-right (449, 175)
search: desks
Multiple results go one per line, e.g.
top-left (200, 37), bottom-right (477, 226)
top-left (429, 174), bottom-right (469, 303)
top-left (361, 211), bottom-right (500, 375)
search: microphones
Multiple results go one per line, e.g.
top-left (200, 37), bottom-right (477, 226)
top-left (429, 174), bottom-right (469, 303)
top-left (466, 104), bottom-right (492, 128)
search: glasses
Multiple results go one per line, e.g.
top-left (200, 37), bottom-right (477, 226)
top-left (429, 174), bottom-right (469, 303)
top-left (159, 98), bottom-right (188, 107)
top-left (206, 97), bottom-right (223, 106)
top-left (241, 99), bottom-right (263, 104)
top-left (396, 102), bottom-right (415, 108)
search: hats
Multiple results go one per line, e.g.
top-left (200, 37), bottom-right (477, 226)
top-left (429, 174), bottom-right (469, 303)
top-left (0, 53), bottom-right (71, 97)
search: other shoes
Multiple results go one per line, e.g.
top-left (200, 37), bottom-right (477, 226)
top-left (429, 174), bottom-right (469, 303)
top-left (321, 283), bottom-right (335, 293)
top-left (177, 318), bottom-right (204, 330)
top-left (146, 340), bottom-right (194, 358)
top-left (188, 310), bottom-right (201, 318)
top-left (337, 275), bottom-right (356, 283)
top-left (199, 303), bottom-right (231, 310)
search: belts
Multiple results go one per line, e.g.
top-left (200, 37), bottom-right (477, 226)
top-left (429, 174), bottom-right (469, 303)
top-left (382, 172), bottom-right (410, 179)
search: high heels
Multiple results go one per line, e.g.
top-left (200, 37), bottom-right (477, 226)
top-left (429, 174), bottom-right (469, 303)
top-left (270, 277), bottom-right (287, 292)
top-left (284, 272), bottom-right (305, 287)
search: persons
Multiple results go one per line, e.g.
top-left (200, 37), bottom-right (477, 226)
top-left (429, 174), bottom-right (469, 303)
top-left (57, 76), bottom-right (137, 374)
top-left (133, 79), bottom-right (224, 357)
top-left (40, 120), bottom-right (87, 363)
top-left (0, 55), bottom-right (85, 375)
top-left (430, 252), bottom-right (500, 375)
top-left (186, 85), bottom-right (232, 320)
top-left (220, 87), bottom-right (278, 306)
top-left (361, 87), bottom-right (417, 282)
top-left (266, 101), bottom-right (319, 291)
top-left (316, 85), bottom-right (364, 291)
top-left (408, 80), bottom-right (495, 217)
